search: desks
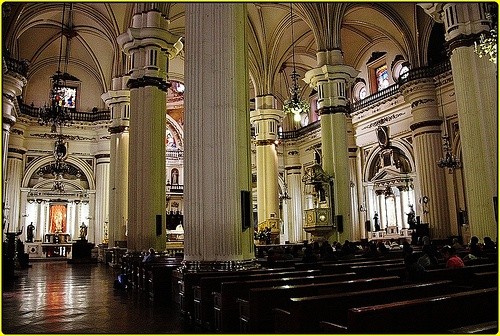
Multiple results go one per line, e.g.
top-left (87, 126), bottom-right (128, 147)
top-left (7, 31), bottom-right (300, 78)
top-left (41, 241), bottom-right (77, 256)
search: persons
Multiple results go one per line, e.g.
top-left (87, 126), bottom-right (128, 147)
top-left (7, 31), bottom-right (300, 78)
top-left (27, 222), bottom-right (35, 243)
top-left (404, 204), bottom-right (416, 229)
top-left (444, 248), bottom-right (465, 268)
top-left (373, 210), bottom-right (381, 232)
top-left (172, 170), bottom-right (178, 184)
top-left (142, 248), bottom-right (154, 262)
top-left (79, 222), bottom-right (87, 238)
top-left (267, 236), bottom-right (497, 275)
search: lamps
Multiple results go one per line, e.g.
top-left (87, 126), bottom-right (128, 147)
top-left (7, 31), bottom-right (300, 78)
top-left (39, 2), bottom-right (73, 131)
top-left (282, 3), bottom-right (310, 122)
top-left (53, 173), bottom-right (66, 194)
top-left (436, 74), bottom-right (461, 174)
top-left (282, 141), bottom-right (292, 205)
top-left (38, 126), bottom-right (79, 176)
top-left (474, 5), bottom-right (497, 64)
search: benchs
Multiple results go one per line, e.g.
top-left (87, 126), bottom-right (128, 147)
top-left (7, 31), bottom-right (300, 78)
top-left (120, 244), bottom-right (497, 334)
